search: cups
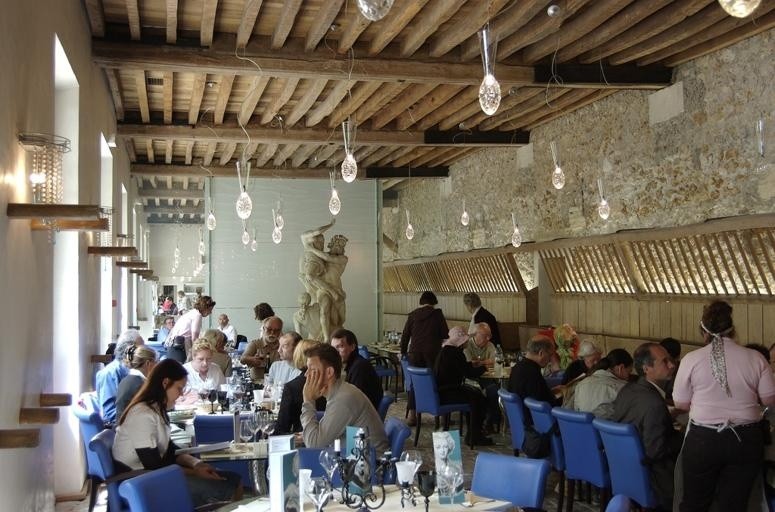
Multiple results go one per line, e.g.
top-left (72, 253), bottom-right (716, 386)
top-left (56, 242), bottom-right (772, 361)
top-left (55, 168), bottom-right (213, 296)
top-left (493, 363), bottom-right (503, 376)
top-left (502, 366), bottom-right (511, 376)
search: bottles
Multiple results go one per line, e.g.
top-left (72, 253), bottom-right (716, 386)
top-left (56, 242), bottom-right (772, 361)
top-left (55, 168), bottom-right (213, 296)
top-left (495, 343), bottom-right (504, 364)
top-left (265, 352), bottom-right (271, 373)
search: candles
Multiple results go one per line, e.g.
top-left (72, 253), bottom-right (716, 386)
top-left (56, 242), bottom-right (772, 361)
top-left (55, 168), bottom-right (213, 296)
top-left (335, 436), bottom-right (342, 452)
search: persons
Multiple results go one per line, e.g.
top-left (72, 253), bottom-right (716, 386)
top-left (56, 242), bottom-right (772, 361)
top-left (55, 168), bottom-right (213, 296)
top-left (399, 290), bottom-right (504, 450)
top-left (97, 290), bottom-right (389, 512)
top-left (506, 298), bottom-right (775, 512)
top-left (292, 220), bottom-right (349, 346)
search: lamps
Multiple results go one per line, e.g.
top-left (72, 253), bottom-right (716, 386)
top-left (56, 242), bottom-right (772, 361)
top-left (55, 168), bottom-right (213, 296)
top-left (95, 207), bottom-right (158, 283)
top-left (170, 226), bottom-right (207, 282)
top-left (719, 0), bottom-right (761, 20)
top-left (475, 30), bottom-right (503, 116)
top-left (16, 130), bottom-right (70, 205)
top-left (356, 0), bottom-right (396, 21)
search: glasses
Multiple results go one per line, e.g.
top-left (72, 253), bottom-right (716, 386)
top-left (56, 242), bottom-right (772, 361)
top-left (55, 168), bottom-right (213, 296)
top-left (264, 325), bottom-right (282, 334)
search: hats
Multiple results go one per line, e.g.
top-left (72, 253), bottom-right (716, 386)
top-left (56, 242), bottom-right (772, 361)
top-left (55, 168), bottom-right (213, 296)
top-left (440, 325), bottom-right (470, 349)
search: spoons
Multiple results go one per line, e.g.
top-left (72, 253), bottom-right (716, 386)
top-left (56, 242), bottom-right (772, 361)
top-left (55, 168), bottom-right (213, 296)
top-left (460, 500), bottom-right (496, 508)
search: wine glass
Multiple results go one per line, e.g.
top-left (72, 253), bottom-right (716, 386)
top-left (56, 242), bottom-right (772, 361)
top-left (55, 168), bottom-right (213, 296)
top-left (256, 410), bottom-right (268, 446)
top-left (399, 450), bottom-right (423, 501)
top-left (304, 478), bottom-right (331, 511)
top-left (416, 470), bottom-right (437, 511)
top-left (246, 413), bottom-right (262, 449)
top-left (218, 391), bottom-right (228, 415)
top-left (207, 383), bottom-right (217, 415)
top-left (238, 419), bottom-right (252, 453)
top-left (198, 383), bottom-right (210, 415)
top-left (440, 462), bottom-right (463, 512)
top-left (318, 449), bottom-right (340, 499)
top-left (261, 415), bottom-right (274, 444)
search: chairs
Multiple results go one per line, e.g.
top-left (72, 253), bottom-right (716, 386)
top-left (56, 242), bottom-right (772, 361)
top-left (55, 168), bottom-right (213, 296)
top-left (192, 413), bottom-right (261, 444)
top-left (236, 334), bottom-right (247, 349)
top-left (300, 341), bottom-right (476, 488)
top-left (471, 388), bottom-right (657, 512)
top-left (145, 340), bottom-right (168, 360)
top-left (72, 397), bottom-right (195, 512)
